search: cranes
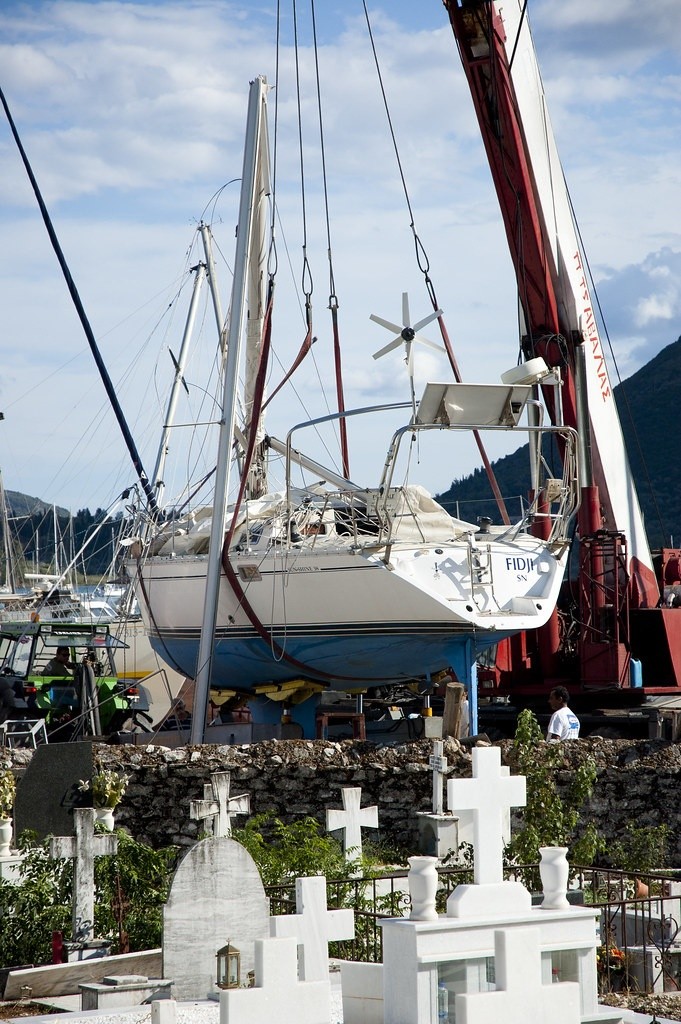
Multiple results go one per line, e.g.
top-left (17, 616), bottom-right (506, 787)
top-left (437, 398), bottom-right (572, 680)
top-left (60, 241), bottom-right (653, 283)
top-left (441, 0), bottom-right (681, 699)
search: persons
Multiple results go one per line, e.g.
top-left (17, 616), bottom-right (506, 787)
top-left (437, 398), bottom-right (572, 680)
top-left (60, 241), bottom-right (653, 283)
top-left (41, 646), bottom-right (74, 676)
top-left (546, 686), bottom-right (580, 741)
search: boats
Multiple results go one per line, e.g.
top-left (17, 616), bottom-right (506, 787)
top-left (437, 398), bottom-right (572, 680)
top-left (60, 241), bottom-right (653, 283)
top-left (2, 573), bottom-right (115, 635)
top-left (123, 82), bottom-right (569, 730)
